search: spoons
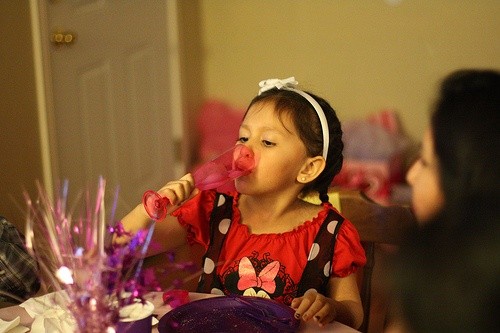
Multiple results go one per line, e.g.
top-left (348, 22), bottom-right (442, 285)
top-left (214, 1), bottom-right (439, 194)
top-left (233, 296), bottom-right (290, 321)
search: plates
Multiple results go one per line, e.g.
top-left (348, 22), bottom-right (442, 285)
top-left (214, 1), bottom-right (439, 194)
top-left (158, 296), bottom-right (300, 333)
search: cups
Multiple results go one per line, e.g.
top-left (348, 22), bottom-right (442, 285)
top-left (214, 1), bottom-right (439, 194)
top-left (115, 298), bottom-right (154, 333)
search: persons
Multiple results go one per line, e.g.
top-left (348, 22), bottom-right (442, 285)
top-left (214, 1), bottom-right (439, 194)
top-left (383, 69), bottom-right (500, 333)
top-left (110, 75), bottom-right (367, 332)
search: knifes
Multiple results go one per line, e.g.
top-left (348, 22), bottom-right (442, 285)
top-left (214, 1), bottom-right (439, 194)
top-left (246, 311), bottom-right (299, 332)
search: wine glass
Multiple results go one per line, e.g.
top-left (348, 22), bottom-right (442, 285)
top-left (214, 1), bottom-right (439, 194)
top-left (142, 145), bottom-right (255, 222)
